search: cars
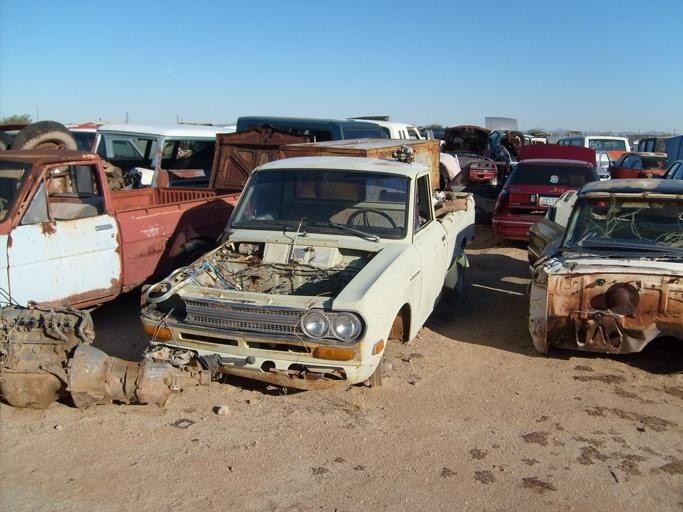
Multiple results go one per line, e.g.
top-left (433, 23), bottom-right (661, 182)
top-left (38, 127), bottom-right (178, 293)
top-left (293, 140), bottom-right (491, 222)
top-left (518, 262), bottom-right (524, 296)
top-left (490, 143), bottom-right (600, 242)
top-left (606, 151), bottom-right (667, 178)
top-left (664, 161), bottom-right (682, 179)
top-left (529, 180), bottom-right (681, 362)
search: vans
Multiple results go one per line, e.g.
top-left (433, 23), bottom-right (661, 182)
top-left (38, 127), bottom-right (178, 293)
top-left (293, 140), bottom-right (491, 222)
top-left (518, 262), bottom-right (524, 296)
top-left (556, 134), bottom-right (630, 181)
top-left (352, 118), bottom-right (423, 141)
top-left (89, 125), bottom-right (234, 190)
top-left (238, 114), bottom-right (388, 144)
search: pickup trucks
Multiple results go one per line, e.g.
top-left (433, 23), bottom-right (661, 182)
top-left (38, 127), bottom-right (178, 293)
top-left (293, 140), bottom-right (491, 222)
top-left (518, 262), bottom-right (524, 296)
top-left (139, 154), bottom-right (476, 393)
top-left (1, 148), bottom-right (242, 316)
